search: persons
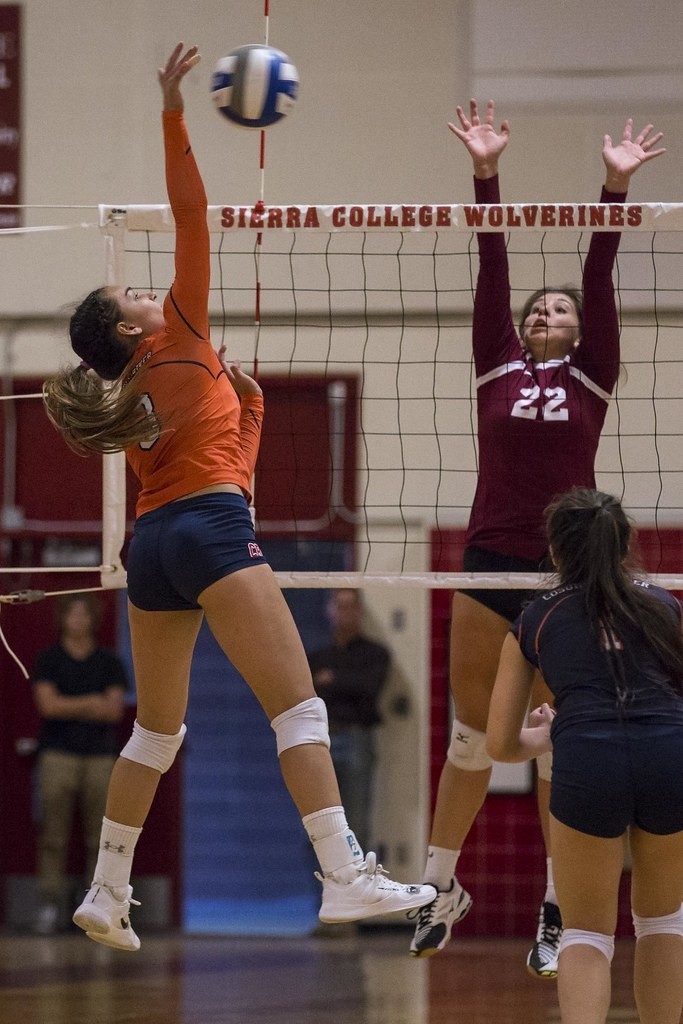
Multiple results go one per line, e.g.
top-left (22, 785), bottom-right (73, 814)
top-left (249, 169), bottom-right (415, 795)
top-left (485, 486), bottom-right (683, 1024)
top-left (408, 97), bottom-right (666, 978)
top-left (306, 587), bottom-right (394, 941)
top-left (42, 39), bottom-right (436, 950)
top-left (30, 591), bottom-right (127, 938)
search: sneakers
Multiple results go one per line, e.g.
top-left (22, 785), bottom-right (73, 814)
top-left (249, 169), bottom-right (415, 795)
top-left (72, 885), bottom-right (142, 952)
top-left (314, 852), bottom-right (437, 924)
top-left (408, 875), bottom-right (473, 960)
top-left (526, 898), bottom-right (564, 979)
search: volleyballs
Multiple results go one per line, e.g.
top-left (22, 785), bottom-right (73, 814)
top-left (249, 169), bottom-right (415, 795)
top-left (206, 44), bottom-right (298, 127)
